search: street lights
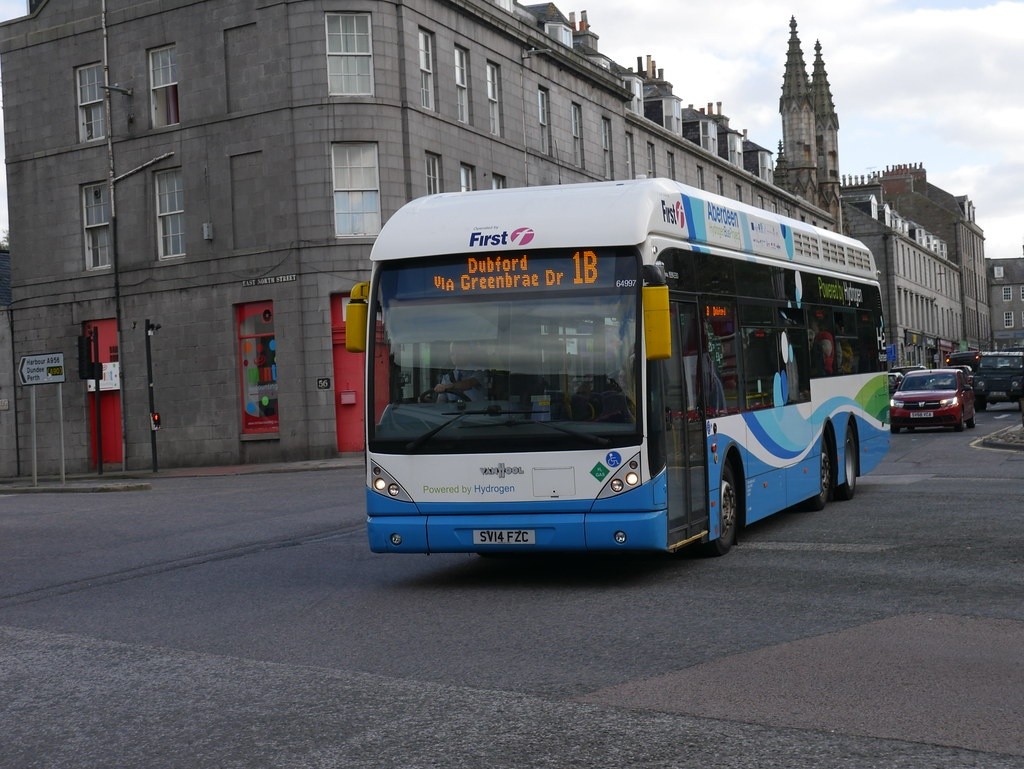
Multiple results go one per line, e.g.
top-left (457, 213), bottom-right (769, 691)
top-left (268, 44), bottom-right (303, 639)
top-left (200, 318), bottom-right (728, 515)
top-left (144, 318), bottom-right (162, 473)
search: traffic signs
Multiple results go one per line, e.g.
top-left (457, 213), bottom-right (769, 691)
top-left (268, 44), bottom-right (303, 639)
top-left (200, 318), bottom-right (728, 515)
top-left (18, 351), bottom-right (66, 386)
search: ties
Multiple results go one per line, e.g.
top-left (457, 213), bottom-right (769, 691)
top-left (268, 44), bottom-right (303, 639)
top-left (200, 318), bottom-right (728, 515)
top-left (457, 369), bottom-right (463, 381)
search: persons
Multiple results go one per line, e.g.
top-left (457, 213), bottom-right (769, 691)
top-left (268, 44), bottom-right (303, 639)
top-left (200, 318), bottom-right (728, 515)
top-left (434, 340), bottom-right (489, 403)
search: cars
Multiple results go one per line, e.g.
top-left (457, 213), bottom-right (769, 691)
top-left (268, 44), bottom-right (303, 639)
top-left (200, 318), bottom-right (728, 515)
top-left (889, 364), bottom-right (929, 403)
top-left (889, 368), bottom-right (977, 433)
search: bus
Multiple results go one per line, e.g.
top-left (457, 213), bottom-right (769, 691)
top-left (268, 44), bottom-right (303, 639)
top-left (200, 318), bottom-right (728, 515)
top-left (346, 176), bottom-right (891, 556)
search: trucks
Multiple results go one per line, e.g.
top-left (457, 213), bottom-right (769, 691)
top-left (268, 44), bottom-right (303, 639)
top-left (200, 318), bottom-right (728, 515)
top-left (942, 351), bottom-right (1024, 418)
